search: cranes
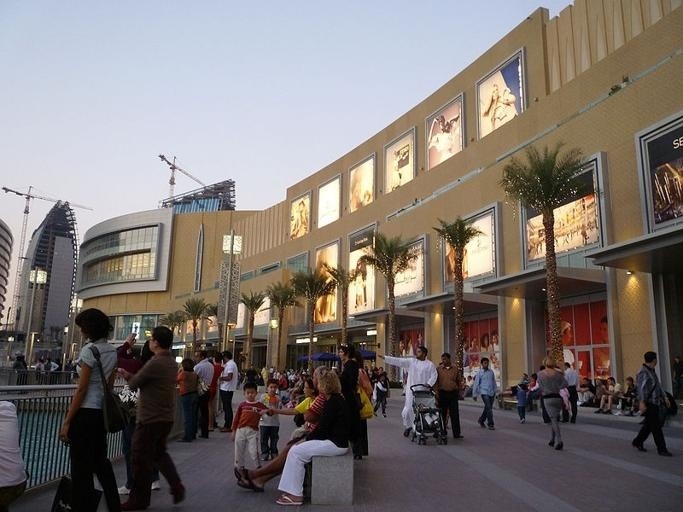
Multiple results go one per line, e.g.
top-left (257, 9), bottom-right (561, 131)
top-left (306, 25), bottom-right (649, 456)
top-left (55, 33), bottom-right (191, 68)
top-left (0, 182), bottom-right (96, 351)
top-left (158, 153), bottom-right (206, 198)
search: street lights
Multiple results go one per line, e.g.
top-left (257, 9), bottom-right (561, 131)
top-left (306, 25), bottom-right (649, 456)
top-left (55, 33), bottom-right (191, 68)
top-left (6, 335), bottom-right (15, 368)
top-left (62, 323), bottom-right (71, 371)
top-left (28, 331), bottom-right (39, 370)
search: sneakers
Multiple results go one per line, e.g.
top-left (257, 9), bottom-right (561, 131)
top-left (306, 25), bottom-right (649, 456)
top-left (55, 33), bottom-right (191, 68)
top-left (174, 425), bottom-right (232, 443)
top-left (260, 452), bottom-right (278, 462)
top-left (151, 479), bottom-right (162, 490)
top-left (657, 448), bottom-right (673, 457)
top-left (120, 500), bottom-right (149, 511)
top-left (478, 418), bottom-right (496, 430)
top-left (170, 484), bottom-right (186, 504)
top-left (403, 427), bottom-right (413, 437)
top-left (631, 438), bottom-right (648, 452)
top-left (118, 485), bottom-right (132, 495)
top-left (237, 479), bottom-right (265, 493)
top-left (593, 408), bottom-right (624, 416)
top-left (233, 464), bottom-right (250, 481)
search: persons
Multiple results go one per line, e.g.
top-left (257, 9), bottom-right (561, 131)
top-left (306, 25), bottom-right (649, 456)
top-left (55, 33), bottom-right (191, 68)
top-left (234, 360), bottom-right (388, 426)
top-left (0, 400), bottom-right (28, 512)
top-left (219, 351), bottom-right (238, 432)
top-left (337, 346), bottom-right (368, 460)
top-left (234, 367), bottom-right (329, 492)
top-left (397, 331), bottom-right (424, 382)
top-left (289, 200), bottom-right (310, 240)
top-left (427, 113), bottom-right (459, 160)
top-left (417, 403), bottom-right (439, 428)
top-left (630, 351), bottom-right (674, 458)
top-left (231, 382), bottom-right (269, 482)
top-left (479, 82), bottom-right (518, 131)
top-left (12, 354), bottom-right (74, 395)
top-left (176, 350), bottom-right (222, 443)
top-left (536, 356), bottom-right (568, 450)
top-left (435, 361), bottom-right (678, 425)
top-left (561, 320), bottom-right (575, 362)
top-left (431, 352), bottom-right (465, 439)
top-left (376, 345), bottom-right (439, 436)
top-left (471, 357), bottom-right (498, 430)
top-left (115, 332), bottom-right (161, 494)
top-left (265, 378), bottom-right (319, 439)
top-left (276, 368), bottom-right (349, 505)
top-left (56, 307), bottom-right (124, 512)
top-left (593, 316), bottom-right (609, 376)
top-left (462, 330), bottom-right (500, 388)
top-left (118, 326), bottom-right (186, 510)
top-left (672, 355), bottom-right (683, 399)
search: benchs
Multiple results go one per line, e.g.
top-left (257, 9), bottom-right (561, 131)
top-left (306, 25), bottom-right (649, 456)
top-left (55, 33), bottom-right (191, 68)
top-left (306, 440), bottom-right (354, 504)
top-left (533, 399), bottom-right (541, 414)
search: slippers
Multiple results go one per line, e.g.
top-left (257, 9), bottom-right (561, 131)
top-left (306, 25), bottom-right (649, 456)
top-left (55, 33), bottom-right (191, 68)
top-left (271, 491), bottom-right (306, 507)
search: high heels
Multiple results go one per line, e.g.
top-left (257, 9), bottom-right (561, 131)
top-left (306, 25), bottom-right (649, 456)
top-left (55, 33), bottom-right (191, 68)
top-left (548, 438), bottom-right (564, 450)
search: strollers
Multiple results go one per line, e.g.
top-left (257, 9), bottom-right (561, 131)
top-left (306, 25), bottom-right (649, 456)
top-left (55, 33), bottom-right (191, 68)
top-left (409, 382), bottom-right (450, 445)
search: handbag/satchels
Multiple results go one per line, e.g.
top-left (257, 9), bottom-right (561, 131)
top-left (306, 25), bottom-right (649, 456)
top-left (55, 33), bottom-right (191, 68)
top-left (102, 390), bottom-right (128, 433)
top-left (355, 385), bottom-right (374, 420)
top-left (358, 368), bottom-right (373, 395)
top-left (51, 474), bottom-right (103, 512)
top-left (197, 377), bottom-right (211, 402)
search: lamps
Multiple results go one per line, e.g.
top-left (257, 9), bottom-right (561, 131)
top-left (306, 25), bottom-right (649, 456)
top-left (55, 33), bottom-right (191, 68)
top-left (627, 270), bottom-right (632, 275)
